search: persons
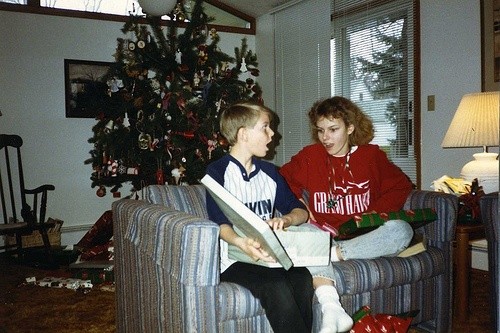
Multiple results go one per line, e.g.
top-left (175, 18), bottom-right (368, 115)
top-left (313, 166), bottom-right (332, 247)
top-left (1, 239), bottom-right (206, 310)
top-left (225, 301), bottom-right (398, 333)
top-left (204, 101), bottom-right (315, 333)
top-left (280, 96), bottom-right (415, 333)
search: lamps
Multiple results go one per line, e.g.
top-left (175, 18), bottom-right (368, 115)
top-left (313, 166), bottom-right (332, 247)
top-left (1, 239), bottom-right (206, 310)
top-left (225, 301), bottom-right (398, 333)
top-left (441, 91), bottom-right (500, 153)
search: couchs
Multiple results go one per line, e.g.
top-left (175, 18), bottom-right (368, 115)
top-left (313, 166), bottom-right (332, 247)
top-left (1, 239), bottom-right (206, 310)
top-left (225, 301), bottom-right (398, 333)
top-left (112, 184), bottom-right (459, 333)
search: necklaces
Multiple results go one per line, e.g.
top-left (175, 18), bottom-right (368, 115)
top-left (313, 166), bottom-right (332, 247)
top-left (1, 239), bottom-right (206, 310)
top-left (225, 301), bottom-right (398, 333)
top-left (327, 144), bottom-right (355, 208)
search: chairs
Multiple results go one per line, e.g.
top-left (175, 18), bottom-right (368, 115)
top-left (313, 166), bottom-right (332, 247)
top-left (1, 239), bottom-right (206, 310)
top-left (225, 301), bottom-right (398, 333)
top-left (0, 134), bottom-right (56, 267)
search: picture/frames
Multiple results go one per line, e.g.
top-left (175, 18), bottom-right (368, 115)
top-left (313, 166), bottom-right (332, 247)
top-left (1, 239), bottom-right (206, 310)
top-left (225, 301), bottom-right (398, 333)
top-left (64, 59), bottom-right (118, 118)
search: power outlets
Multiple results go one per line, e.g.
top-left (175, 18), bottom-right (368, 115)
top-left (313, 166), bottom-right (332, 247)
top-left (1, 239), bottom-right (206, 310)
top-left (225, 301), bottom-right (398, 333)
top-left (428, 96), bottom-right (436, 111)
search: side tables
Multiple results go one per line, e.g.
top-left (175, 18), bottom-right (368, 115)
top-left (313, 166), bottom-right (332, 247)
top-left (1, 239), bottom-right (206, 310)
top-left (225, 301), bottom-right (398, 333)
top-left (451, 217), bottom-right (485, 333)
